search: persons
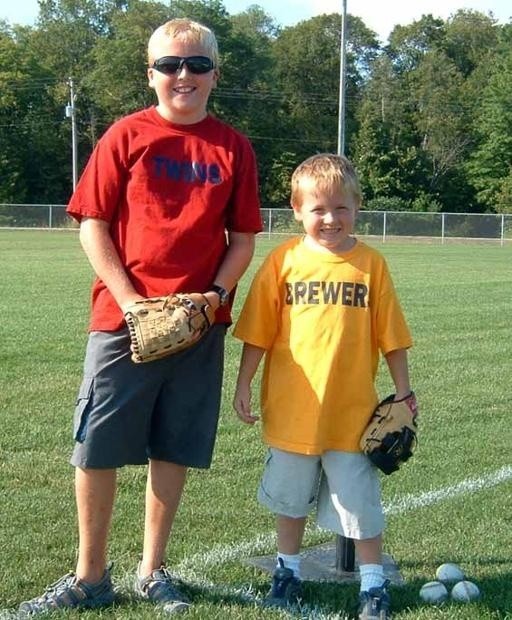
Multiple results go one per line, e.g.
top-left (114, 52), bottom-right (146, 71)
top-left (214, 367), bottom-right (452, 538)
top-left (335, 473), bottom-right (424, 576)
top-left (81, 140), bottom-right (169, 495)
top-left (19, 19), bottom-right (263, 614)
top-left (233, 153), bottom-right (414, 620)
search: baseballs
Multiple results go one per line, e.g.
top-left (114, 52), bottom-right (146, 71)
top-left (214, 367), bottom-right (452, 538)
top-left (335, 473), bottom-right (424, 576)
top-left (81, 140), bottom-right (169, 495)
top-left (452, 580), bottom-right (479, 602)
top-left (437, 562), bottom-right (463, 584)
top-left (420, 582), bottom-right (449, 603)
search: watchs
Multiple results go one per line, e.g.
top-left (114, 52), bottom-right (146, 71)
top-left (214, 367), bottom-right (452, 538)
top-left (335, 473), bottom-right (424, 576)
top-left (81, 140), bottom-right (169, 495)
top-left (208, 286), bottom-right (229, 307)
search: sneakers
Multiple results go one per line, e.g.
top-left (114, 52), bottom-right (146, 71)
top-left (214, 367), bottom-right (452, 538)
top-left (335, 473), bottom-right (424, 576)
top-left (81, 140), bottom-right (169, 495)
top-left (266, 568), bottom-right (302, 611)
top-left (136, 561), bottom-right (194, 617)
top-left (18, 564), bottom-right (117, 617)
top-left (358, 586), bottom-right (389, 620)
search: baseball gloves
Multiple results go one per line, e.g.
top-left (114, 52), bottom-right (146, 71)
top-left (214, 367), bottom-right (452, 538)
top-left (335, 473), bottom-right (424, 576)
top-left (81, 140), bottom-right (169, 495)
top-left (123, 291), bottom-right (221, 365)
top-left (359, 389), bottom-right (418, 473)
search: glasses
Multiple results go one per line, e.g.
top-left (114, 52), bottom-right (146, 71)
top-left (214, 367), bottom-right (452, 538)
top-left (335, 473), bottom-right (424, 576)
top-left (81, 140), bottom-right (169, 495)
top-left (153, 56), bottom-right (215, 74)
